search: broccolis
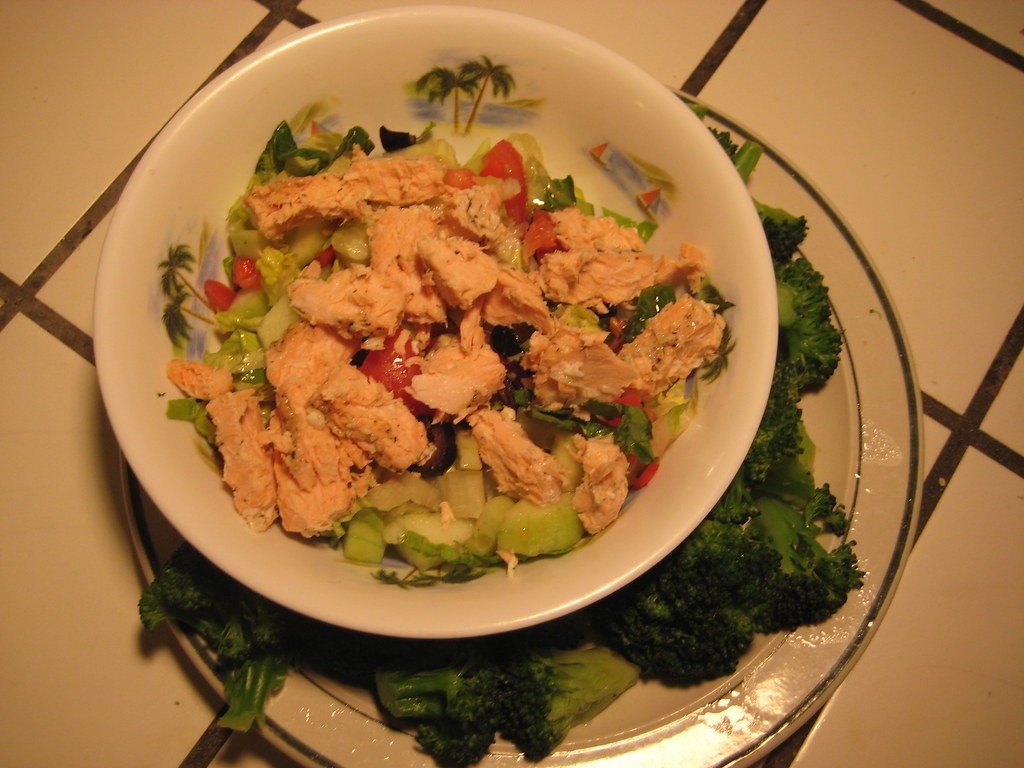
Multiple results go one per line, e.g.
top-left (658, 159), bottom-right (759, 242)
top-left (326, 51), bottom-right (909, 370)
top-left (686, 105), bottom-right (846, 406)
top-left (136, 394), bottom-right (868, 768)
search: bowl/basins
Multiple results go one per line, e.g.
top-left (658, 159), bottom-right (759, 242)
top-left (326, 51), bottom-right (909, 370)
top-left (92, 6), bottom-right (777, 638)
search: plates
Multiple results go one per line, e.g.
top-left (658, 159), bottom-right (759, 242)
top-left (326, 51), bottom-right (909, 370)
top-left (119, 83), bottom-right (923, 768)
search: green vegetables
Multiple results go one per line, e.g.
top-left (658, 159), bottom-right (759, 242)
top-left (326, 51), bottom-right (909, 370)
top-left (156, 116), bottom-right (734, 574)
